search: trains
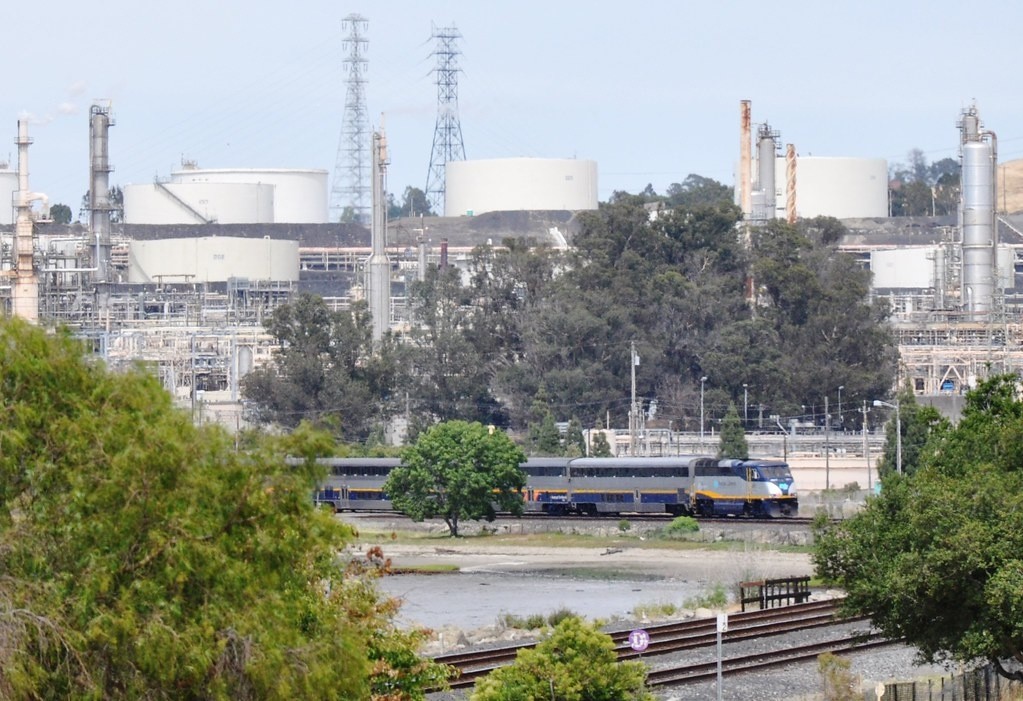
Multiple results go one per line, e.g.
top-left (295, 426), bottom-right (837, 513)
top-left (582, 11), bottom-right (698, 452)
top-left (289, 455), bottom-right (801, 522)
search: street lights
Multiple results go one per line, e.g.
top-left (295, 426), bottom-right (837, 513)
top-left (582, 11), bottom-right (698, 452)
top-left (630, 354), bottom-right (640, 455)
top-left (742, 384), bottom-right (750, 431)
top-left (873, 401), bottom-right (902, 477)
top-left (700, 376), bottom-right (709, 455)
top-left (838, 385), bottom-right (844, 428)
